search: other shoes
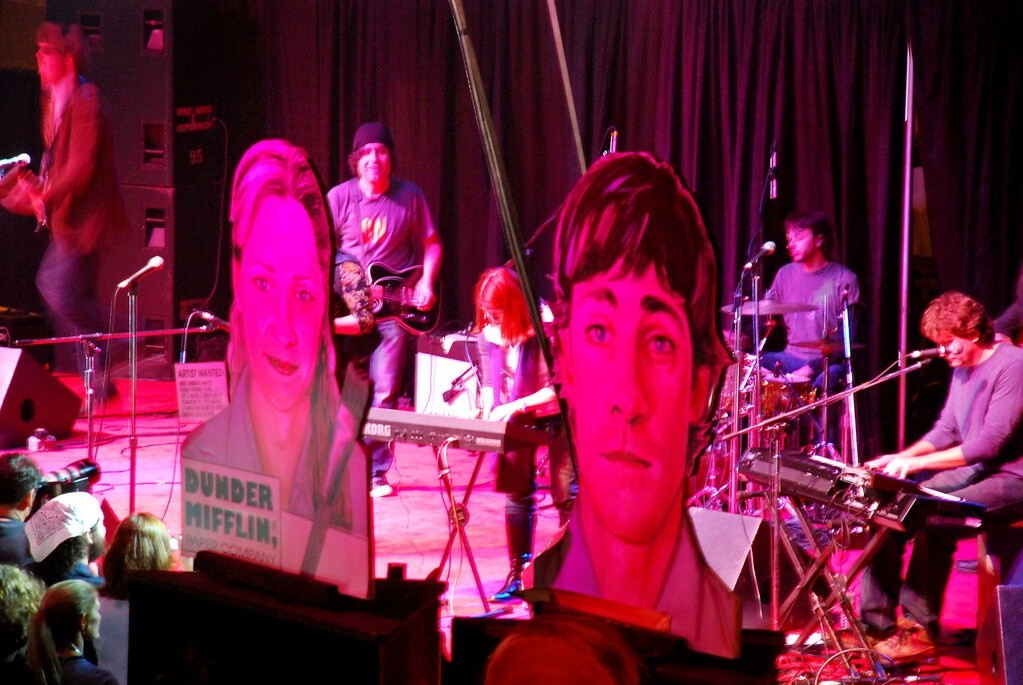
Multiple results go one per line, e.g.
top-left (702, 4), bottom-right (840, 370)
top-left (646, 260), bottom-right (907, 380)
top-left (829, 627), bottom-right (892, 652)
top-left (367, 475), bottom-right (393, 497)
top-left (79, 380), bottom-right (116, 414)
top-left (861, 616), bottom-right (936, 667)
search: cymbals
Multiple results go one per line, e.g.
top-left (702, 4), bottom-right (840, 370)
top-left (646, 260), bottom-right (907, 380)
top-left (793, 338), bottom-right (866, 352)
top-left (720, 300), bottom-right (820, 316)
top-left (732, 351), bottom-right (763, 363)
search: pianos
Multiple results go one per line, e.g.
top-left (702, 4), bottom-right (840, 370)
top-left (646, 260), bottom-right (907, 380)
top-left (360, 407), bottom-right (564, 616)
top-left (736, 452), bottom-right (986, 684)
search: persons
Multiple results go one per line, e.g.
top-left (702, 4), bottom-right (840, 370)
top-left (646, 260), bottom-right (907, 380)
top-left (520, 151), bottom-right (741, 659)
top-left (0, 453), bottom-right (171, 683)
top-left (36, 20), bottom-right (120, 417)
top-left (480, 614), bottom-right (645, 685)
top-left (762, 208), bottom-right (862, 448)
top-left (180, 140), bottom-right (373, 603)
top-left (469, 265), bottom-right (560, 601)
top-left (822, 272), bottom-right (1023, 667)
top-left (325, 121), bottom-right (444, 496)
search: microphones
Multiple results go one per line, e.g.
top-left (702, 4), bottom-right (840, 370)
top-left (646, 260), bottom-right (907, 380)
top-left (0, 153), bottom-right (31, 172)
top-left (743, 241), bottom-right (776, 270)
top-left (770, 144), bottom-right (779, 199)
top-left (117, 256), bottom-right (163, 288)
top-left (197, 311), bottom-right (231, 335)
top-left (610, 130), bottom-right (618, 153)
top-left (912, 340), bottom-right (964, 359)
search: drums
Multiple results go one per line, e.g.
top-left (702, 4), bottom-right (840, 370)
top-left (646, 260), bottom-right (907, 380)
top-left (717, 362), bottom-right (778, 413)
top-left (756, 373), bottom-right (811, 418)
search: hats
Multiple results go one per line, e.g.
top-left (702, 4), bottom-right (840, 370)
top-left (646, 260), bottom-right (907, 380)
top-left (353, 121), bottom-right (395, 153)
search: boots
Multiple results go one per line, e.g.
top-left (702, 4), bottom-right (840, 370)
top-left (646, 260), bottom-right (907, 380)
top-left (488, 503), bottom-right (537, 602)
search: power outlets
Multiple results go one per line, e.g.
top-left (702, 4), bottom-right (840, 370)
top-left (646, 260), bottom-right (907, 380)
top-left (194, 105), bottom-right (215, 130)
top-left (175, 106), bottom-right (194, 132)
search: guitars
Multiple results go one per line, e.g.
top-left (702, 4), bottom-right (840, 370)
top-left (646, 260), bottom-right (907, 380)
top-left (0, 163), bottom-right (53, 228)
top-left (360, 261), bottom-right (445, 336)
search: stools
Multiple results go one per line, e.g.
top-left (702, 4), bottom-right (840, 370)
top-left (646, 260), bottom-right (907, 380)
top-left (974, 519), bottom-right (1023, 685)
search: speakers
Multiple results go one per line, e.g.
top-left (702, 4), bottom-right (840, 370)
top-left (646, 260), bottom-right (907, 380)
top-left (688, 507), bottom-right (839, 629)
